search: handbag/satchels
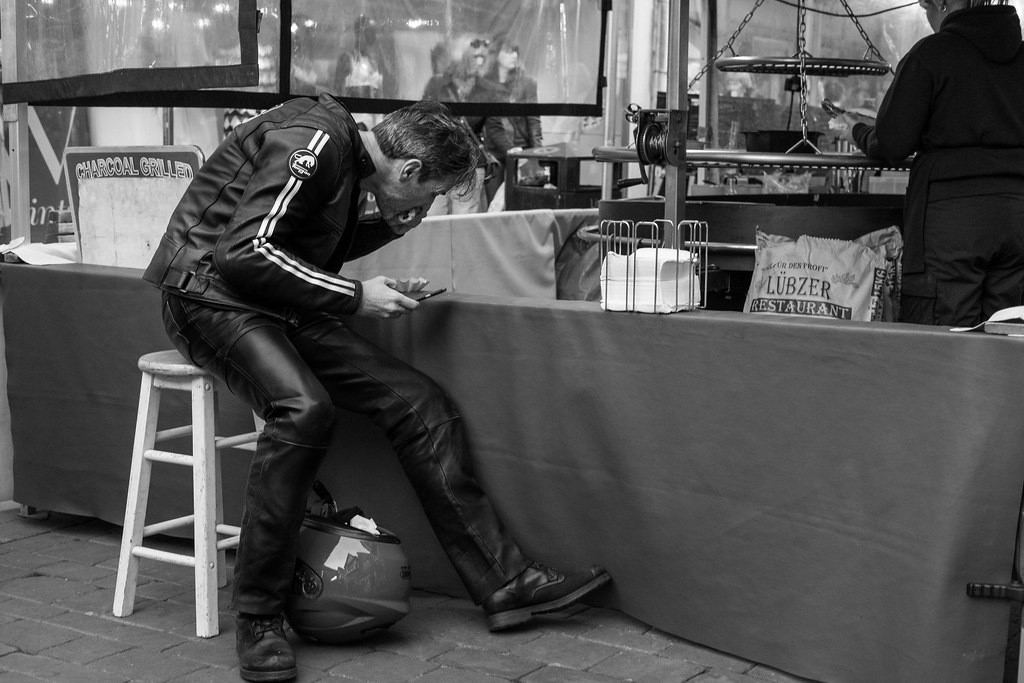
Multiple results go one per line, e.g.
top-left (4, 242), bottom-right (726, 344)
top-left (518, 154), bottom-right (550, 187)
top-left (484, 151), bottom-right (503, 204)
top-left (743, 224), bottom-right (904, 322)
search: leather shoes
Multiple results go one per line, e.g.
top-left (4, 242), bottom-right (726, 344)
top-left (482, 561), bottom-right (611, 631)
top-left (235, 615), bottom-right (297, 682)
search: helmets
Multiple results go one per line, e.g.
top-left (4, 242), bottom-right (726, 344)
top-left (283, 506), bottom-right (411, 646)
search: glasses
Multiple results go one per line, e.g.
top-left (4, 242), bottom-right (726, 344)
top-left (470, 38), bottom-right (491, 48)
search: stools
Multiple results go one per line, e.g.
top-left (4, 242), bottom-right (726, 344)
top-left (112, 348), bottom-right (269, 639)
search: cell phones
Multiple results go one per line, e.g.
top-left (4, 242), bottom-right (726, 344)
top-left (416, 288), bottom-right (447, 301)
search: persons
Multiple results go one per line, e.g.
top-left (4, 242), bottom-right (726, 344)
top-left (421, 34), bottom-right (522, 217)
top-left (482, 36), bottom-right (543, 207)
top-left (334, 14), bottom-right (388, 98)
top-left (837, 0), bottom-right (1024, 327)
top-left (291, 15), bottom-right (317, 95)
top-left (142, 93), bottom-right (611, 680)
